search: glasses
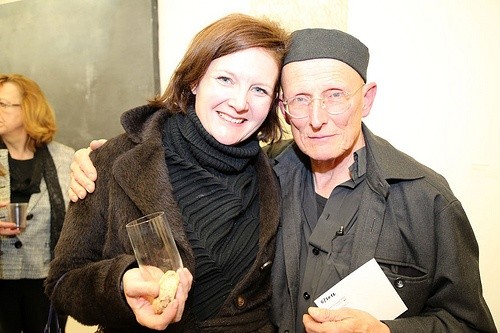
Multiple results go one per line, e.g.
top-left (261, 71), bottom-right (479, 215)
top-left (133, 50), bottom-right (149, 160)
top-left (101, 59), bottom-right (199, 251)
top-left (282, 82), bottom-right (365, 118)
top-left (0, 99), bottom-right (21, 110)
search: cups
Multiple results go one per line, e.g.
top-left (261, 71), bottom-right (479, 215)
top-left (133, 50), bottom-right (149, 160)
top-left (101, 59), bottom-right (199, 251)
top-left (126, 212), bottom-right (183, 281)
top-left (7, 203), bottom-right (28, 230)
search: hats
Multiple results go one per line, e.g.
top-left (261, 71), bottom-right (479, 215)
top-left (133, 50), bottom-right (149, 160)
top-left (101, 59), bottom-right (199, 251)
top-left (284, 28), bottom-right (370, 84)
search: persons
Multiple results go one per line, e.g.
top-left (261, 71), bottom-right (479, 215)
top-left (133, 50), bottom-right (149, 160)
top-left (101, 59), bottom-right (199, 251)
top-left (68, 28), bottom-right (497, 333)
top-left (45, 13), bottom-right (292, 333)
top-left (0, 74), bottom-right (75, 333)
